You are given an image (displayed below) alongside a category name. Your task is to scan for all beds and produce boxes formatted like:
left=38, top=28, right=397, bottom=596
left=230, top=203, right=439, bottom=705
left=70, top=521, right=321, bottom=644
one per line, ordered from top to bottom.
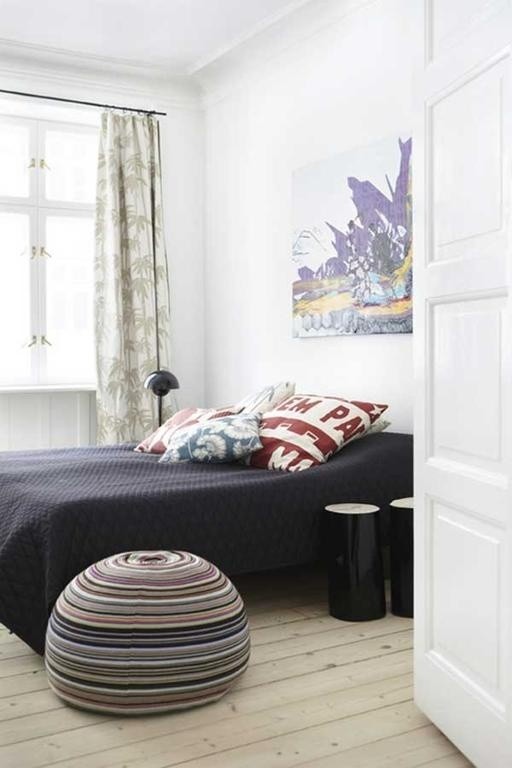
left=0, top=424, right=413, bottom=651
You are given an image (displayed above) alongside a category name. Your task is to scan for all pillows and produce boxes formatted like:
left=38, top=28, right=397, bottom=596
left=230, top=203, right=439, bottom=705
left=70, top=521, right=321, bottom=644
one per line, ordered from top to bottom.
left=233, top=391, right=388, bottom=471
left=135, top=405, right=243, bottom=455
left=159, top=411, right=262, bottom=463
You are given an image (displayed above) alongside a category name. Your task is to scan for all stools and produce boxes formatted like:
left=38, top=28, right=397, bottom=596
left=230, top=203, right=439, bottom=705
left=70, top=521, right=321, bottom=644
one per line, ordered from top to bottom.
left=45, top=548, right=252, bottom=718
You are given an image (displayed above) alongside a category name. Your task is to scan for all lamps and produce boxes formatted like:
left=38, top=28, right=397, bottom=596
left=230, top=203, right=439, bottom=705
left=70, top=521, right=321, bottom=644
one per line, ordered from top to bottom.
left=143, top=370, right=179, bottom=427
